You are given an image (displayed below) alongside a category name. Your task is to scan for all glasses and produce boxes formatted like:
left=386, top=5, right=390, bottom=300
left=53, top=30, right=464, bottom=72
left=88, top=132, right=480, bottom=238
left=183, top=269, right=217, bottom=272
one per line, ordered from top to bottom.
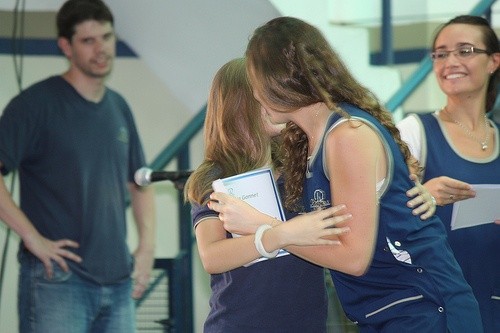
left=430, top=46, right=489, bottom=62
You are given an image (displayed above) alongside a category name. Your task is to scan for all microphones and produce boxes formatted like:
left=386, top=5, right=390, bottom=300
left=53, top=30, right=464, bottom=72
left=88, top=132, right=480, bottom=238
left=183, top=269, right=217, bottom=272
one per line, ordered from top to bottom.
left=134, top=167, right=194, bottom=187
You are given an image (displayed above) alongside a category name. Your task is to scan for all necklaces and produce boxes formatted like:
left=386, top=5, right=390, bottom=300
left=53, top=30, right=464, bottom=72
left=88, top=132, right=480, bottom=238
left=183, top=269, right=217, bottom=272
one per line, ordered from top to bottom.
left=306, top=102, right=325, bottom=160
left=443, top=105, right=492, bottom=151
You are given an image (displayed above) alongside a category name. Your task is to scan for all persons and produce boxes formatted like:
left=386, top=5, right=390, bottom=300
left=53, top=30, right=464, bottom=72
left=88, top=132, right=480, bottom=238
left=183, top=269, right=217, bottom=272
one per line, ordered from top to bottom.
left=0, top=0, right=160, bottom=333
left=395, top=15, right=500, bottom=333
left=205, top=16, right=484, bottom=332
left=182, top=58, right=438, bottom=333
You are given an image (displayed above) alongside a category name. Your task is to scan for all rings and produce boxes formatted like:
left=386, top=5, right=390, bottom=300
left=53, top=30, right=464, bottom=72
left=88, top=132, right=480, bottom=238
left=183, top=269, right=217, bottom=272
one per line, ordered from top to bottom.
left=450, top=194, right=456, bottom=203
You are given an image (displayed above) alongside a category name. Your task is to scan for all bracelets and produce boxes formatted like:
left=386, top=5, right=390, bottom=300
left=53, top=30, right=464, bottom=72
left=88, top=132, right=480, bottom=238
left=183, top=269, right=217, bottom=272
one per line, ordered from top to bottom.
left=270, top=216, right=277, bottom=227
left=254, top=223, right=280, bottom=259
